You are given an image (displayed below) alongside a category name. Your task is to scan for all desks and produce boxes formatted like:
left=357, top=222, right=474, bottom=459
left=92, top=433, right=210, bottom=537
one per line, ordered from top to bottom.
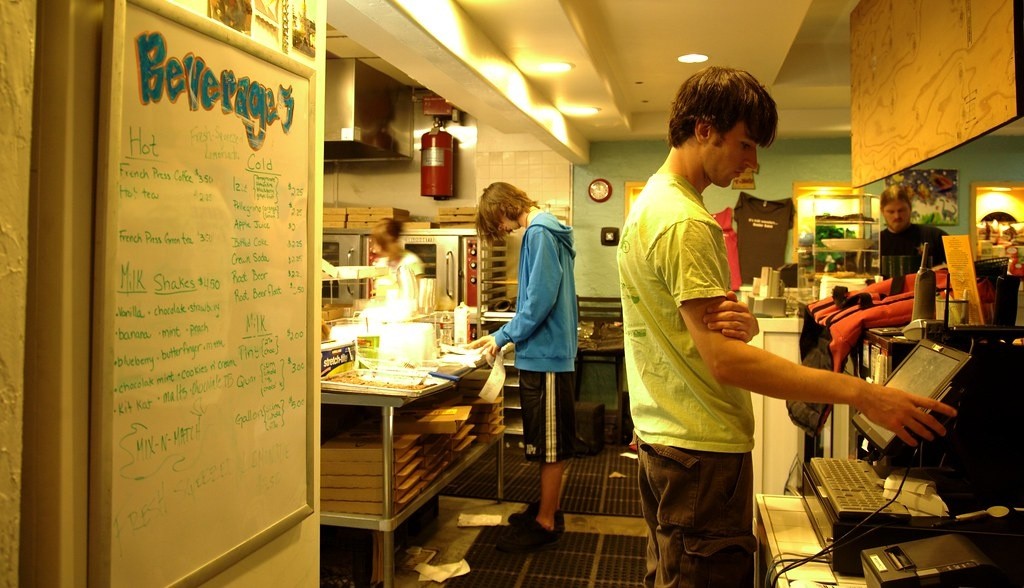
left=320, top=341, right=516, bottom=588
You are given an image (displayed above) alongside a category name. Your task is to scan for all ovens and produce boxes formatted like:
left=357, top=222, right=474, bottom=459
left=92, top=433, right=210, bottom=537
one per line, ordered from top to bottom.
left=323, top=227, right=499, bottom=343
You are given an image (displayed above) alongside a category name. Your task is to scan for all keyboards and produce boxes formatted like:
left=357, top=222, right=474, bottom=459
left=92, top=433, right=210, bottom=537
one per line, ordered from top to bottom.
left=810, top=458, right=911, bottom=523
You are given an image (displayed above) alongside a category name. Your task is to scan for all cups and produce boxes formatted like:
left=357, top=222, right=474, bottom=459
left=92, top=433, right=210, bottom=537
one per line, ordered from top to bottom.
left=991, top=275, right=1021, bottom=327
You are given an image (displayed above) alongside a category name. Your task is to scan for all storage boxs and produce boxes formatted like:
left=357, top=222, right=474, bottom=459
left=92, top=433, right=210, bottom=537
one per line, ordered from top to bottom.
left=320, top=368, right=507, bottom=514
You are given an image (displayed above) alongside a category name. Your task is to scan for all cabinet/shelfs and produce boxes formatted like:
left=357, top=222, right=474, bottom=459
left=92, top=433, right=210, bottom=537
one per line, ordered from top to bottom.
left=795, top=194, right=881, bottom=291
left=477, top=203, right=571, bottom=448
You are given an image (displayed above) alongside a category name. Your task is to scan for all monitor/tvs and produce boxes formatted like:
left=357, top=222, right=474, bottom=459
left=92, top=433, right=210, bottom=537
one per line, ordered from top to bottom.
left=852, top=339, right=973, bottom=457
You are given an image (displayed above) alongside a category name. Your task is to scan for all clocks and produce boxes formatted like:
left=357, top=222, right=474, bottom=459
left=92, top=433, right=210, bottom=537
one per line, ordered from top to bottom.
left=589, top=179, right=612, bottom=202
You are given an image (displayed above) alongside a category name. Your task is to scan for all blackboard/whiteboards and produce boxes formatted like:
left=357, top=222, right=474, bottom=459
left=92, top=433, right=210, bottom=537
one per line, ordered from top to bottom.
left=93, top=0, right=320, bottom=588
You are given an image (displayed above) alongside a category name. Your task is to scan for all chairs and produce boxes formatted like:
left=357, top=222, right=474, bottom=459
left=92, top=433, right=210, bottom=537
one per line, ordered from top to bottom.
left=572, top=294, right=626, bottom=398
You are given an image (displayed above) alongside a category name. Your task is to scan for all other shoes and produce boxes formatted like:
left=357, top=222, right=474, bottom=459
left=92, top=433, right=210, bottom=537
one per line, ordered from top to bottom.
left=508, top=506, right=564, bottom=534
left=496, top=517, right=561, bottom=555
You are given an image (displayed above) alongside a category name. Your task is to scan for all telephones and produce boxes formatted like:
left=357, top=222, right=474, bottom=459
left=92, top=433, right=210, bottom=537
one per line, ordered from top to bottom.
left=904, top=241, right=946, bottom=340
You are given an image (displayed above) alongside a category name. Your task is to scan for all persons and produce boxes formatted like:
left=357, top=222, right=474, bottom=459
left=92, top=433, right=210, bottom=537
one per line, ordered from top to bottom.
left=616, top=68, right=956, bottom=588
left=464, top=182, right=579, bottom=553
left=869, top=184, right=950, bottom=272
left=371, top=220, right=425, bottom=310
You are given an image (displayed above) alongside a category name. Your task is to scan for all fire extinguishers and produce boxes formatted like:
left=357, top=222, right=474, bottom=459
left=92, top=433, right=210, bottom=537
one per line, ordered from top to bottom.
left=417, top=113, right=455, bottom=199
